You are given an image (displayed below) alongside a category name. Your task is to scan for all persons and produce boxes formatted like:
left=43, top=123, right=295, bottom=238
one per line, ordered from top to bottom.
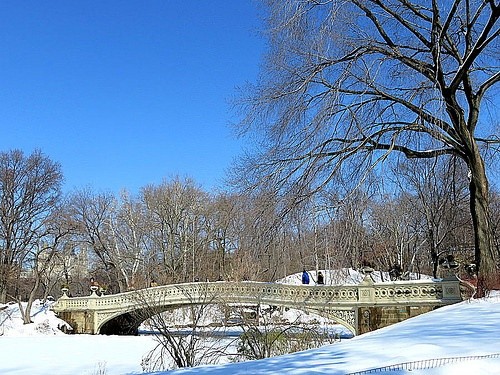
left=302, top=270, right=309, bottom=284
left=318, top=272, right=324, bottom=284
